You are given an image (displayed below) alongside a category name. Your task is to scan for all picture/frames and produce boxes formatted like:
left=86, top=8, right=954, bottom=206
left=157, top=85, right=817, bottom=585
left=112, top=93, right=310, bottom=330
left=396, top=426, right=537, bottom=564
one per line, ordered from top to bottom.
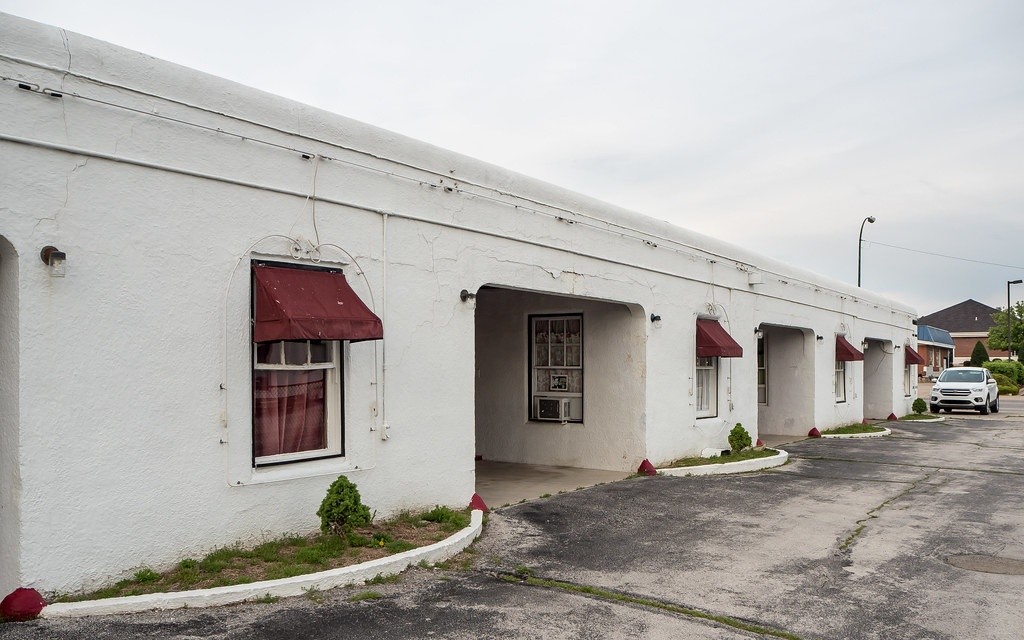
left=550, top=375, right=568, bottom=391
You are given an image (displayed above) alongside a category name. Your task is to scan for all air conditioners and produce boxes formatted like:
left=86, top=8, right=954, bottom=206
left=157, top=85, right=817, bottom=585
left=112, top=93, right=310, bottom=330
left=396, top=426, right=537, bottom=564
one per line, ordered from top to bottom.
left=537, top=398, right=571, bottom=420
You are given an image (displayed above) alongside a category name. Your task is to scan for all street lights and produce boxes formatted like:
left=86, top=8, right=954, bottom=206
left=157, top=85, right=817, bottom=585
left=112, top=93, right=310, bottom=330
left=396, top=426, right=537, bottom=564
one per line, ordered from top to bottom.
left=857, top=216, right=876, bottom=287
left=1007, top=280, right=1023, bottom=364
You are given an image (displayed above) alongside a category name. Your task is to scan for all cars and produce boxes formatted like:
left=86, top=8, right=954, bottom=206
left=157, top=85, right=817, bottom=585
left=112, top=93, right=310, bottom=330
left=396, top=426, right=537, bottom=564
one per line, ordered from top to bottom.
left=930, top=366, right=1000, bottom=415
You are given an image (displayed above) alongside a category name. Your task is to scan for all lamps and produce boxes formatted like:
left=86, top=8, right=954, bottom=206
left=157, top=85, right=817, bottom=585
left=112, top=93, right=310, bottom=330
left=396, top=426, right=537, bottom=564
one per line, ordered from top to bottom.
left=42, top=246, right=68, bottom=278
left=461, top=289, right=476, bottom=313
left=650, top=314, right=660, bottom=328
left=754, top=326, right=764, bottom=338
left=817, top=334, right=824, bottom=345
left=861, top=341, right=869, bottom=350
left=894, top=345, right=902, bottom=353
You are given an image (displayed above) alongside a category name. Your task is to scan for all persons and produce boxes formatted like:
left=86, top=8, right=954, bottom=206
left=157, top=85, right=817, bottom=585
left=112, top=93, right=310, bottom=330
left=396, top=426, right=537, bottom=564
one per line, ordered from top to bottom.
left=552, top=378, right=563, bottom=389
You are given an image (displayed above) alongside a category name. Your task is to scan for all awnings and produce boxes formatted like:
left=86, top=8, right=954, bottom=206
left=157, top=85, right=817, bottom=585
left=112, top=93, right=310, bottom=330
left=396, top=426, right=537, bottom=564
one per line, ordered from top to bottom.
left=252, top=264, right=385, bottom=342
left=697, top=319, right=744, bottom=358
left=836, top=336, right=865, bottom=361
left=905, top=346, right=925, bottom=364
